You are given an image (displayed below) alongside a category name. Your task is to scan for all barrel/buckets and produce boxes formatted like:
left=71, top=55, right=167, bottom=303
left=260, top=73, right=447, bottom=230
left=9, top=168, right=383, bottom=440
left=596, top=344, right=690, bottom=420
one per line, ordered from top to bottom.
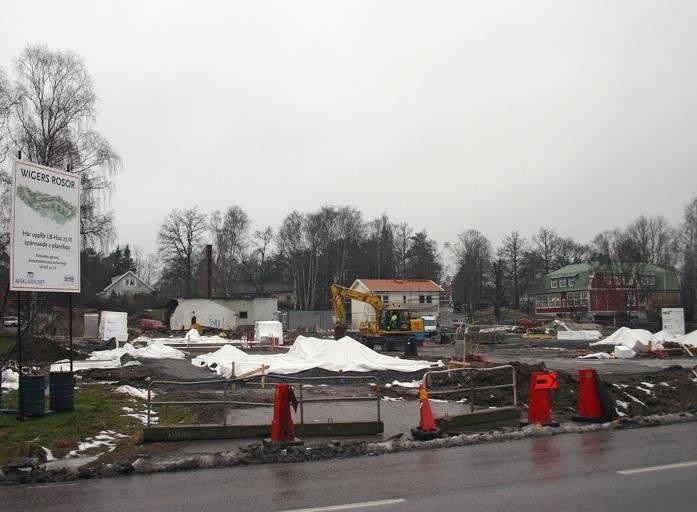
left=85, top=314, right=98, bottom=338
left=455, top=339, right=473, bottom=359
left=49, top=371, right=74, bottom=411
left=19, top=373, right=45, bottom=417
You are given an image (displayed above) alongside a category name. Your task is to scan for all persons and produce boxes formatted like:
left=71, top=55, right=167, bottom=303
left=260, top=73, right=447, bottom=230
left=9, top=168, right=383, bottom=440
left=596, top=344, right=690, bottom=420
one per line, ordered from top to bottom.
left=191, top=309, right=196, bottom=325
left=390, top=310, right=397, bottom=322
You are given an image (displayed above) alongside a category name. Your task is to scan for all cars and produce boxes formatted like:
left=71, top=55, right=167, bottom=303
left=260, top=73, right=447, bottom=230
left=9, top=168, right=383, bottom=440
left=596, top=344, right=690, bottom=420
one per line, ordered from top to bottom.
left=51, top=305, right=100, bottom=317
left=138, top=317, right=166, bottom=332
left=478, top=324, right=554, bottom=335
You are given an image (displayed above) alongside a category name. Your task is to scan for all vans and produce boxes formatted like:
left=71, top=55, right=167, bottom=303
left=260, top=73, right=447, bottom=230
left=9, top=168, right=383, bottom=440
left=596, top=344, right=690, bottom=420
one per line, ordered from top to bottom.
left=418, top=315, right=443, bottom=336
left=3, top=316, right=27, bottom=326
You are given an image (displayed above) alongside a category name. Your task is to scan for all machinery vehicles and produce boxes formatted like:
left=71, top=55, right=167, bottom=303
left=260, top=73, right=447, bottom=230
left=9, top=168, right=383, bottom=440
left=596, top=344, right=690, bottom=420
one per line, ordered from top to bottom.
left=328, top=278, right=426, bottom=355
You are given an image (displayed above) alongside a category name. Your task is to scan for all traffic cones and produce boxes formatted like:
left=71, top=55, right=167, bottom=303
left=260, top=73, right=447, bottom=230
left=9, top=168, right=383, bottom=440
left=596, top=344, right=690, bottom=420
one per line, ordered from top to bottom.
left=572, top=365, right=607, bottom=424
left=518, top=369, right=562, bottom=427
left=261, top=382, right=304, bottom=450
left=414, top=383, right=439, bottom=433
